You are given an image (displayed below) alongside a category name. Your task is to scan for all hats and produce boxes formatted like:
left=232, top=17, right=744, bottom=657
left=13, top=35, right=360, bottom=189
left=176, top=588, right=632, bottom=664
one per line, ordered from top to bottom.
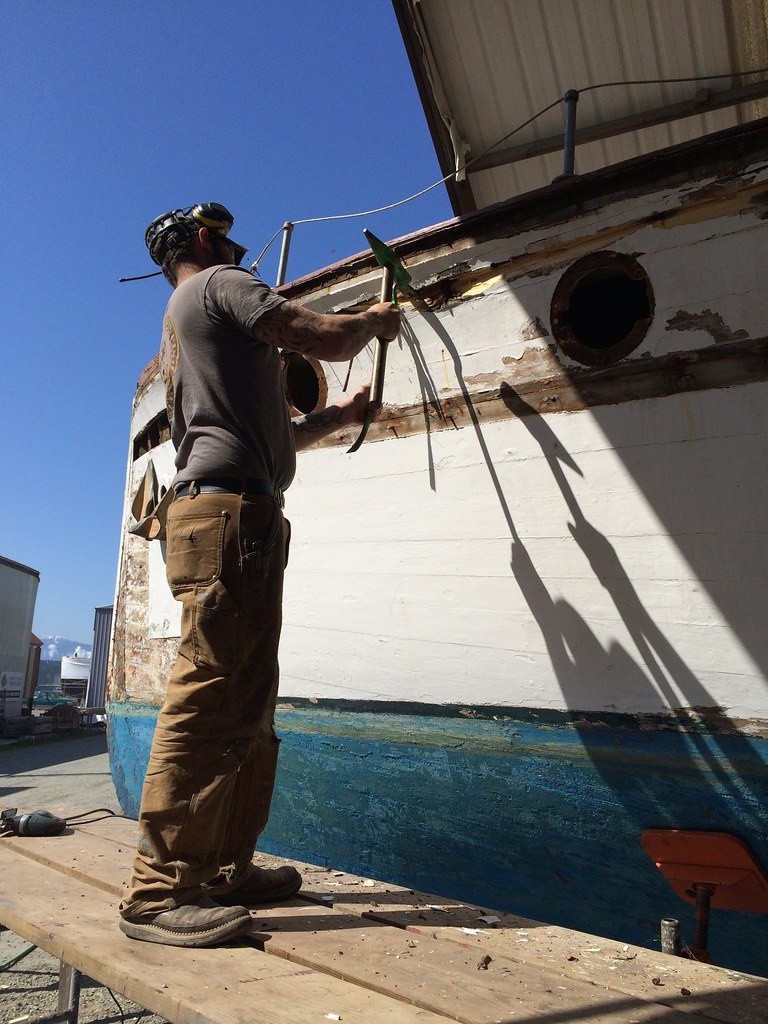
left=146, top=214, right=248, bottom=267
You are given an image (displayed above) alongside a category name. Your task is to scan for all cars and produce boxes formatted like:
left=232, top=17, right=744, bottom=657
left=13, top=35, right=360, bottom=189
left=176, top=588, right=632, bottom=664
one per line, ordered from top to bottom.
left=22, top=690, right=76, bottom=711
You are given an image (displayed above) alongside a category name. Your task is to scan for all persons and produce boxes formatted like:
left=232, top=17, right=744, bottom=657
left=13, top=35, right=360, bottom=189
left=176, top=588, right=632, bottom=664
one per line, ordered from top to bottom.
left=116, top=201, right=400, bottom=947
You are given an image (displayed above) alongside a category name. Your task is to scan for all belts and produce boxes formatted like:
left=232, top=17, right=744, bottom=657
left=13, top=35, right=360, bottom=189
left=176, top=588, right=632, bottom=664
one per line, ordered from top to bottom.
left=172, top=477, right=285, bottom=510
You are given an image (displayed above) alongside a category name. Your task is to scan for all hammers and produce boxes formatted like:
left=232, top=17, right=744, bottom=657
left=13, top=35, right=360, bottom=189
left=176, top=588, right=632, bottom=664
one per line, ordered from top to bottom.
left=362, top=227, right=413, bottom=410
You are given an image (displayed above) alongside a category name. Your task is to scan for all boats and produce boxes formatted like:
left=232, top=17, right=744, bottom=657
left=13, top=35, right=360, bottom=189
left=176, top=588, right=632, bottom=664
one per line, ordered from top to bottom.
left=105, top=2, right=767, bottom=980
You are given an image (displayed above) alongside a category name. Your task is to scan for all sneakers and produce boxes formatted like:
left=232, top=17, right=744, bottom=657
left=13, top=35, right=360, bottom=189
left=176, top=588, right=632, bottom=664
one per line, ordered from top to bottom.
left=205, top=865, right=303, bottom=906
left=121, top=900, right=255, bottom=946
